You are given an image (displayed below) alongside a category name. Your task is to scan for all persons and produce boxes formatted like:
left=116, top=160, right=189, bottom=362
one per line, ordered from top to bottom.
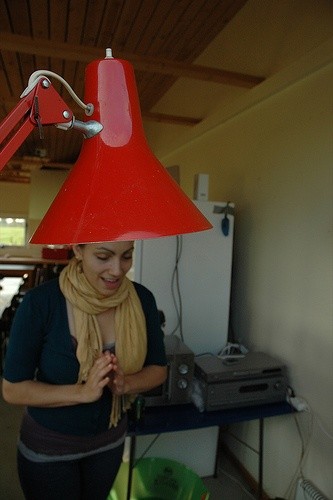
left=0, top=241, right=168, bottom=500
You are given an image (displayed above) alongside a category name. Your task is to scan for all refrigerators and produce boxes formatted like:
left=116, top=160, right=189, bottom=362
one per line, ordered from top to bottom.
left=121, top=201, right=235, bottom=477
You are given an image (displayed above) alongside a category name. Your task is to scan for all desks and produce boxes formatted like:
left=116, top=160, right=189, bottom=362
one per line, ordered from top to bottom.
left=125, top=401, right=304, bottom=500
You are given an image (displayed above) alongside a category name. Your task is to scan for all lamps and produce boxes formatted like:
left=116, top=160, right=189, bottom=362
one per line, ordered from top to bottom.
left=0, top=48, right=213, bottom=245
